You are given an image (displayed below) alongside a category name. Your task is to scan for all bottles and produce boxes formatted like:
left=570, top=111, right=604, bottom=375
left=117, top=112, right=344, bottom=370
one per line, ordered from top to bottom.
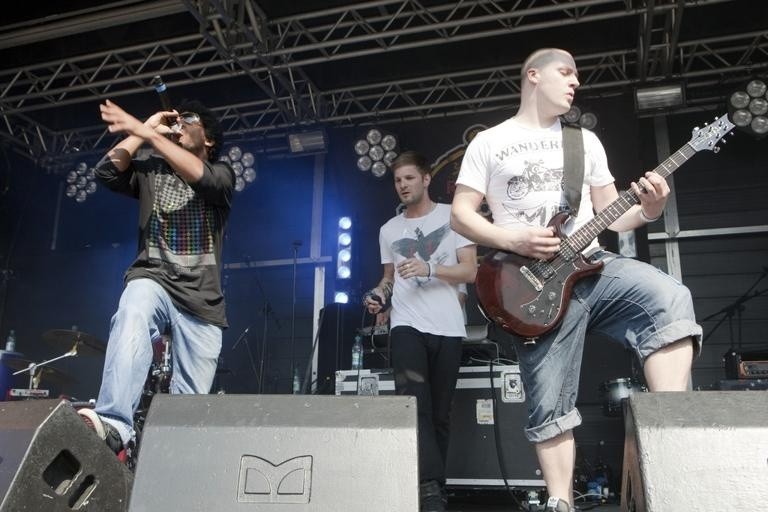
left=5, top=330, right=17, bottom=352
left=350, top=336, right=365, bottom=370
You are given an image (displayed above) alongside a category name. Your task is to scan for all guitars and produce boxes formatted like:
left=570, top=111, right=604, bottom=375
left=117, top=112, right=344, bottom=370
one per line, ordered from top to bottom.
left=475, top=113, right=737, bottom=345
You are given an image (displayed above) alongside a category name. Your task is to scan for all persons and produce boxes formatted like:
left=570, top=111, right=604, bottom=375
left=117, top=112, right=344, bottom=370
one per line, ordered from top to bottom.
left=446, top=45, right=704, bottom=511
left=75, top=97, right=239, bottom=465
left=358, top=147, right=481, bottom=511
left=452, top=281, right=470, bottom=329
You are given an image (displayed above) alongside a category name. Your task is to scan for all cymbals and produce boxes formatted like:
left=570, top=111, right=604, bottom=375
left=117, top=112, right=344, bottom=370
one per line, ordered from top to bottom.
left=41, top=329, right=107, bottom=361
left=4, top=357, right=81, bottom=388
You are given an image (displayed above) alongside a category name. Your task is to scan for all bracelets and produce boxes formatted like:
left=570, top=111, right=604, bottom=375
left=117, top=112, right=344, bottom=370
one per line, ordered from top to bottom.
left=638, top=210, right=663, bottom=224
left=426, top=261, right=433, bottom=280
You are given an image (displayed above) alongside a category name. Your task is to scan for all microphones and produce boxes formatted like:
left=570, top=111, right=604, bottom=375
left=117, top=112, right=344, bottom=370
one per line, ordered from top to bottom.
left=153, top=71, right=183, bottom=138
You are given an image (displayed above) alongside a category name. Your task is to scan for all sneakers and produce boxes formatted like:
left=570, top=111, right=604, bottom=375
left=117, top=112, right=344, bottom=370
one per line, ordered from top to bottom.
left=78, top=407, right=124, bottom=455
left=421, top=481, right=447, bottom=512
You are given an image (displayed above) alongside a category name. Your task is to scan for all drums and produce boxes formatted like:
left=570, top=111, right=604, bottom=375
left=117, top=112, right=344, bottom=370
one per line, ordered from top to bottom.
left=150, top=334, right=172, bottom=384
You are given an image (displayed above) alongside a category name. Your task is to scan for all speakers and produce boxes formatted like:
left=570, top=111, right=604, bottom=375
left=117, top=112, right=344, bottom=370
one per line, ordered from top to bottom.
left=620, top=387, right=768, bottom=512
left=128, top=394, right=422, bottom=512
left=0, top=397, right=134, bottom=512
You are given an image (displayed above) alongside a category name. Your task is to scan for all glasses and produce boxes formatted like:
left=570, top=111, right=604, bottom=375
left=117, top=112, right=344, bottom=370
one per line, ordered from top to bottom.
left=179, top=112, right=204, bottom=128
